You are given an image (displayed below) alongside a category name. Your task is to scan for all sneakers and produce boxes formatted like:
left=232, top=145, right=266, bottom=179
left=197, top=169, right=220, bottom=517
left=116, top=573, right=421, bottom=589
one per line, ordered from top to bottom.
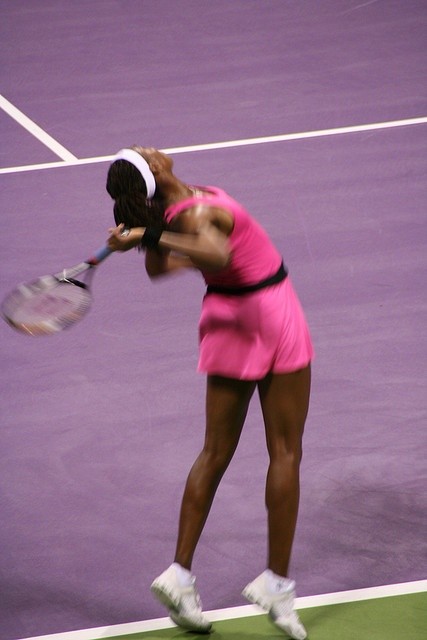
left=149, top=568, right=212, bottom=632
left=241, top=571, right=307, bottom=640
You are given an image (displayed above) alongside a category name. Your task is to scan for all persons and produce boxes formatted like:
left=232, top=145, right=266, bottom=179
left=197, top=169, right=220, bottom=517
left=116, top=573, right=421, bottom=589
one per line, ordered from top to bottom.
left=107, top=146, right=314, bottom=640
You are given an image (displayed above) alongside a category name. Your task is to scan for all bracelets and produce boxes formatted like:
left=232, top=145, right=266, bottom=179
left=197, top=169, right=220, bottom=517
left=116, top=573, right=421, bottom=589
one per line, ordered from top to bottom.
left=140, top=225, right=166, bottom=251
